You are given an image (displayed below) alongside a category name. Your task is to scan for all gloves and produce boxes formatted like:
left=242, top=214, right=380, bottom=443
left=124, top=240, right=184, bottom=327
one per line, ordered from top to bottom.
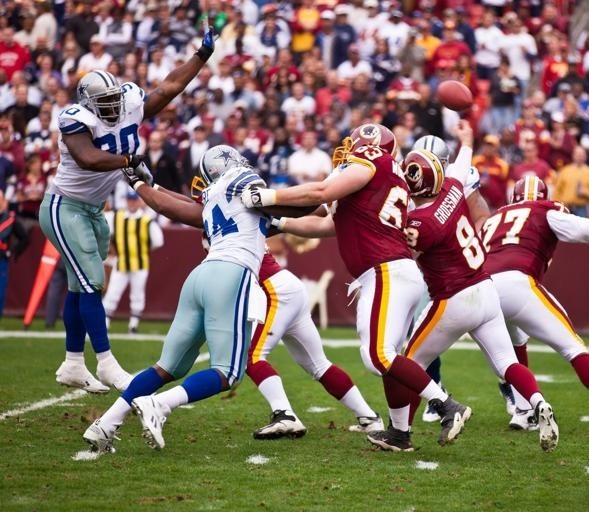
left=194, top=16, right=219, bottom=60
left=121, top=152, right=153, bottom=189
left=241, top=187, right=277, bottom=209
left=265, top=215, right=286, bottom=238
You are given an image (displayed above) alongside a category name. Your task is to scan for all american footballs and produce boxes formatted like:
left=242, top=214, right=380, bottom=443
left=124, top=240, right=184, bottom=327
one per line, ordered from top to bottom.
left=437, top=80, right=473, bottom=111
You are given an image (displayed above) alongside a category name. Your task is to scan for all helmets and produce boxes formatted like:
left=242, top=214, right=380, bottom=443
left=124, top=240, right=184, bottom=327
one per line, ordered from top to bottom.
left=78, top=70, right=126, bottom=125
left=333, top=123, right=450, bottom=197
left=201, top=145, right=248, bottom=183
left=514, top=175, right=547, bottom=202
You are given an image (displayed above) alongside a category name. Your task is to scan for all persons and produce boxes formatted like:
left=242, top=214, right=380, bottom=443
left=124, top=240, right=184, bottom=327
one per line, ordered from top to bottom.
left=1, top=0, right=589, bottom=224
left=37, top=27, right=219, bottom=394
left=393, top=119, right=562, bottom=454
left=241, top=123, right=472, bottom=454
left=407, top=135, right=519, bottom=423
left=84, top=144, right=345, bottom=450
left=124, top=163, right=386, bottom=441
left=472, top=175, right=589, bottom=432
left=104, top=188, right=164, bottom=332
left=0, top=191, right=29, bottom=316
left=44, top=259, right=68, bottom=327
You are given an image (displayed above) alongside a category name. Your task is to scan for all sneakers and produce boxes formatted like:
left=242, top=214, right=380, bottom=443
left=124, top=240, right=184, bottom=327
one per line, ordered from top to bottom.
left=96, top=357, right=133, bottom=392
left=56, top=362, right=111, bottom=393
left=497, top=382, right=559, bottom=454
left=349, top=388, right=471, bottom=454
left=131, top=395, right=165, bottom=450
left=253, top=410, right=307, bottom=438
left=82, top=419, right=116, bottom=454
